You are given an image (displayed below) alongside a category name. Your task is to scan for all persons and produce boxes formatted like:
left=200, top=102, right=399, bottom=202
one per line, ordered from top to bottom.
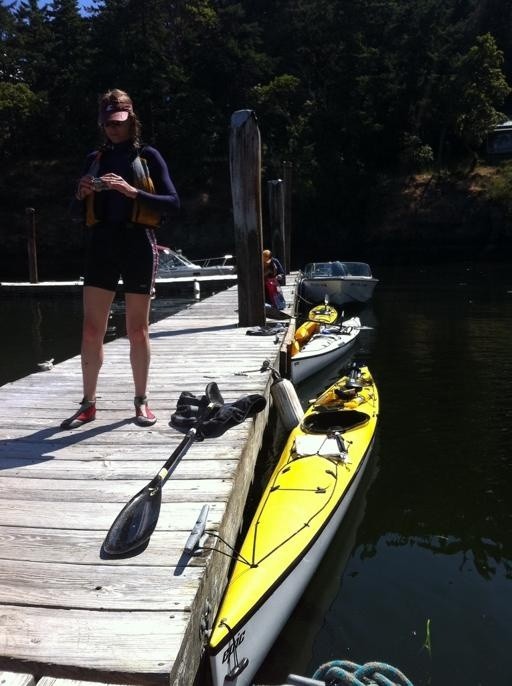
left=58, top=87, right=182, bottom=431
left=263, top=250, right=285, bottom=305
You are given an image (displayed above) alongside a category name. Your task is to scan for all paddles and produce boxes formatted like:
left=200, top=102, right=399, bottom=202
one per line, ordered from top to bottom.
left=324, top=294, right=329, bottom=312
left=305, top=318, right=373, bottom=330
left=101, top=382, right=223, bottom=553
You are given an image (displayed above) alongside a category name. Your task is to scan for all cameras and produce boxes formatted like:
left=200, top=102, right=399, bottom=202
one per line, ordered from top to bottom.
left=92, top=178, right=108, bottom=192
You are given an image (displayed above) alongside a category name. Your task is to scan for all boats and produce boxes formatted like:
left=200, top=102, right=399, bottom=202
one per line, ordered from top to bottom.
left=156, top=244, right=236, bottom=278
left=301, top=261, right=380, bottom=307
left=292, top=304, right=362, bottom=385
left=203, top=364, right=381, bottom=686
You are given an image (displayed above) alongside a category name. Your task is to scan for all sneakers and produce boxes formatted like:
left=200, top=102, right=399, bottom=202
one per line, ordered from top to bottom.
left=133, top=396, right=158, bottom=426
left=59, top=397, right=97, bottom=429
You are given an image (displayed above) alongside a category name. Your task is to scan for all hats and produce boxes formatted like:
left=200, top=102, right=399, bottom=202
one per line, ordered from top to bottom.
left=96, top=99, right=133, bottom=127
left=263, top=249, right=272, bottom=265
